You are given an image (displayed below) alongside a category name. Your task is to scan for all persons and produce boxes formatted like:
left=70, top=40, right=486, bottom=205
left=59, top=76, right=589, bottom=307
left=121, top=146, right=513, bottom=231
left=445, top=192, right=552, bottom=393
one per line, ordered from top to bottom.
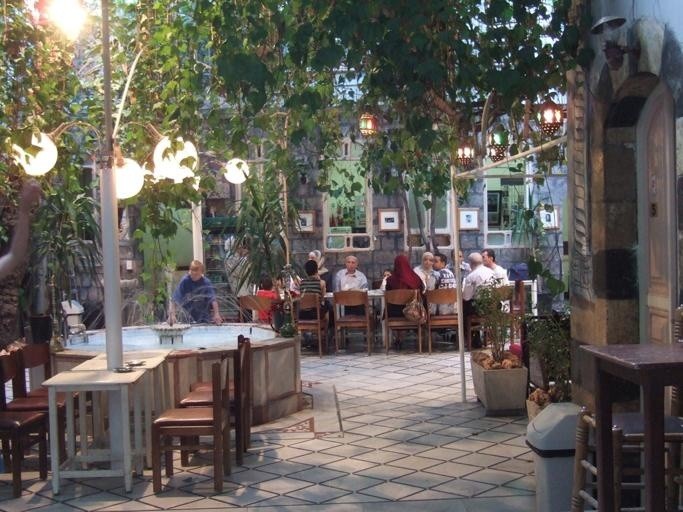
left=222, top=235, right=254, bottom=322
left=308, top=249, right=331, bottom=287
left=379, top=249, right=512, bottom=353
left=332, top=255, right=367, bottom=314
left=165, top=260, right=224, bottom=327
left=258, top=260, right=329, bottom=347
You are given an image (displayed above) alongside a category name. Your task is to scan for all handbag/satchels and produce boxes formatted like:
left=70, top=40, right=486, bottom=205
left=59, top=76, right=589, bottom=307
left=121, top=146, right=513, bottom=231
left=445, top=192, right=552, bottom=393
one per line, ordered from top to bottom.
left=403, top=290, right=428, bottom=325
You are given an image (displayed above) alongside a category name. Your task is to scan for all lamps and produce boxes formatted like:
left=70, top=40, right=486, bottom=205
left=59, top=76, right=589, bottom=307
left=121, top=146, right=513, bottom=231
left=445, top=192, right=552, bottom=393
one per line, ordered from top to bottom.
left=591, top=16, right=641, bottom=71
left=538, top=93, right=564, bottom=138
left=357, top=113, right=378, bottom=137
left=486, top=133, right=505, bottom=163
left=458, top=141, right=474, bottom=167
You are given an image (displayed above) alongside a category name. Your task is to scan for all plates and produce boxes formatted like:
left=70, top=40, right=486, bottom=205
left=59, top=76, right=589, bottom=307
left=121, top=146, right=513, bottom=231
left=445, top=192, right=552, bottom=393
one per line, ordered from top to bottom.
left=126, top=361, right=146, bottom=366
left=112, top=368, right=134, bottom=372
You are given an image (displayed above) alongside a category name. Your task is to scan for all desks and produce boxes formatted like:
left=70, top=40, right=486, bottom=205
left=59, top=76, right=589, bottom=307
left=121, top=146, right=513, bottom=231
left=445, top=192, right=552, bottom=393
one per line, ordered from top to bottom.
left=576, top=342, right=683, bottom=512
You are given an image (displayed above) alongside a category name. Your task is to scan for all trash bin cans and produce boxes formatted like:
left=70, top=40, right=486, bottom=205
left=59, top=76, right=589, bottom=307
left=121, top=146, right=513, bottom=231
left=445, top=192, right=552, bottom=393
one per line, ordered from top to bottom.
left=525, top=402, right=593, bottom=512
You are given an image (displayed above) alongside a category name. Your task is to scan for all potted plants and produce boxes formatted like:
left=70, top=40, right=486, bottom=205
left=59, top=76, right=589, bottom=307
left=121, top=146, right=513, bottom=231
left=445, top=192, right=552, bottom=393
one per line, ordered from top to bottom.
left=470, top=277, right=530, bottom=411
left=526, top=317, right=571, bottom=425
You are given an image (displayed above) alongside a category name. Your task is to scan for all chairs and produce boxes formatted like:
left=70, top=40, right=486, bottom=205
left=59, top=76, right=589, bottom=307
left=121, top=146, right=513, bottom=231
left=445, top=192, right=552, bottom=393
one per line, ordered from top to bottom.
left=238, top=269, right=527, bottom=358
left=571, top=302, right=683, bottom=512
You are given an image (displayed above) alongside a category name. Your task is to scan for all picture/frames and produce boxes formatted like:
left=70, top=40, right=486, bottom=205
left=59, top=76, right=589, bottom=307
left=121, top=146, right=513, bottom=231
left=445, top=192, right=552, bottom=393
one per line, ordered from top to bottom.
left=488, top=190, right=502, bottom=226
left=538, top=205, right=560, bottom=230
left=378, top=208, right=402, bottom=233
left=457, top=208, right=479, bottom=232
left=293, top=210, right=316, bottom=234
